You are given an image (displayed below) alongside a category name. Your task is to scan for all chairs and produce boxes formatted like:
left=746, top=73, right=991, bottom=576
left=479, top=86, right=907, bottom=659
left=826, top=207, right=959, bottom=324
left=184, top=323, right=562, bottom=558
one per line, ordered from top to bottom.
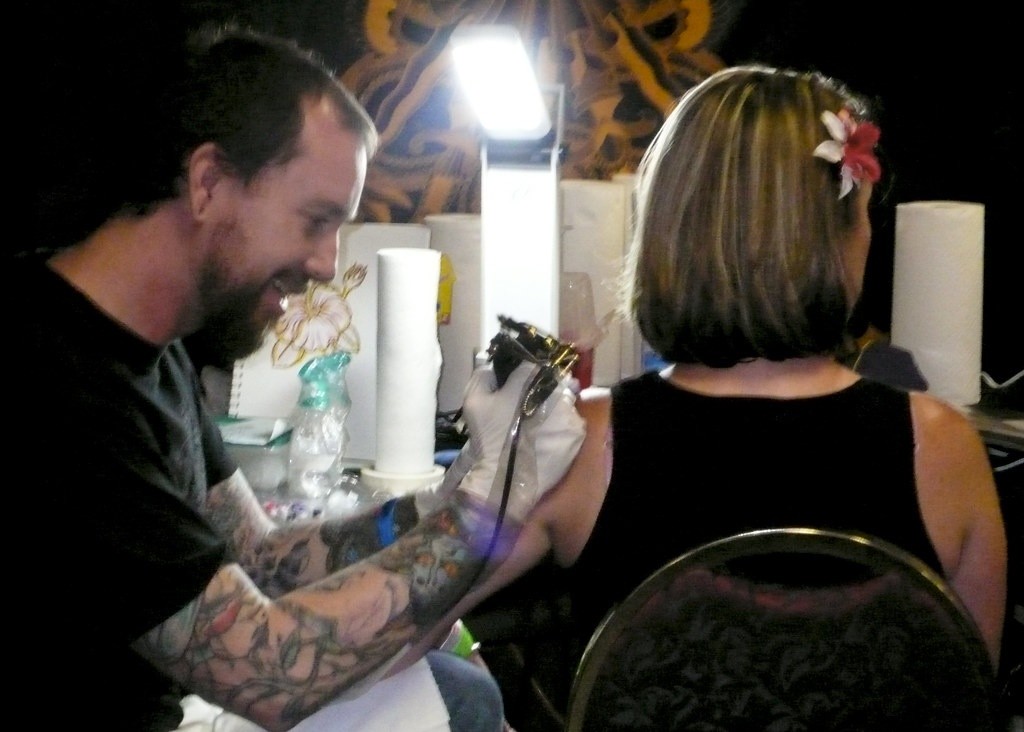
left=470, top=528, right=1014, bottom=732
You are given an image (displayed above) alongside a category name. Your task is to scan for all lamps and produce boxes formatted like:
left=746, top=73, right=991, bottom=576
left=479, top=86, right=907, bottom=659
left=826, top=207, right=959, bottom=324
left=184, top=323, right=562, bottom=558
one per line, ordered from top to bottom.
left=447, top=20, right=566, bottom=345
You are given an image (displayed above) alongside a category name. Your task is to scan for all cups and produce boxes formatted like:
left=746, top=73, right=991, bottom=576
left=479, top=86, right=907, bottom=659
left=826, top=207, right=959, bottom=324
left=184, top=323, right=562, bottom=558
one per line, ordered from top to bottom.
left=558, top=272, right=597, bottom=389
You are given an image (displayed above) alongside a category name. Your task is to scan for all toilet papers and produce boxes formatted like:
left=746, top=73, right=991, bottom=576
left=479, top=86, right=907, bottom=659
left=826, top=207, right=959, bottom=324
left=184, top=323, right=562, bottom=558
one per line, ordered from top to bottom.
left=557, top=170, right=651, bottom=388
left=890, top=200, right=985, bottom=405
left=422, top=211, right=481, bottom=414
left=370, top=246, right=444, bottom=476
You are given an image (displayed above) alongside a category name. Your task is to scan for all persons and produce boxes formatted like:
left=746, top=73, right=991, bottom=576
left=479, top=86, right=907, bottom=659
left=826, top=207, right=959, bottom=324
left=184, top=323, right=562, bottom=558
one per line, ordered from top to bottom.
left=0, top=24, right=587, bottom=732
left=381, top=67, right=1009, bottom=732
